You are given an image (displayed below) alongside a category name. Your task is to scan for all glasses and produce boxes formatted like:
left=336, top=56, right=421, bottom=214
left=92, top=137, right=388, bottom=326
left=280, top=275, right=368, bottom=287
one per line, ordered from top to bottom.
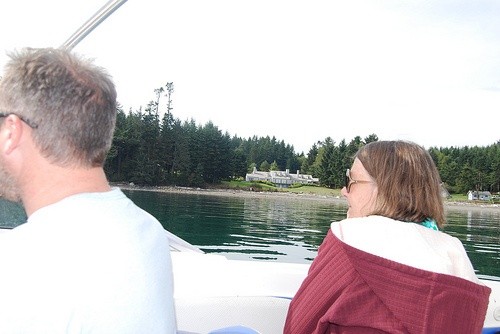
left=346, top=169, right=374, bottom=193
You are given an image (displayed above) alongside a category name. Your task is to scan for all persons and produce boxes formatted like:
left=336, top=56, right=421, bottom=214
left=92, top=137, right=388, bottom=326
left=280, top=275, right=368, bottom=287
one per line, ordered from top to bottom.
left=0, top=48, right=176, bottom=334
left=282, top=141, right=491, bottom=334
